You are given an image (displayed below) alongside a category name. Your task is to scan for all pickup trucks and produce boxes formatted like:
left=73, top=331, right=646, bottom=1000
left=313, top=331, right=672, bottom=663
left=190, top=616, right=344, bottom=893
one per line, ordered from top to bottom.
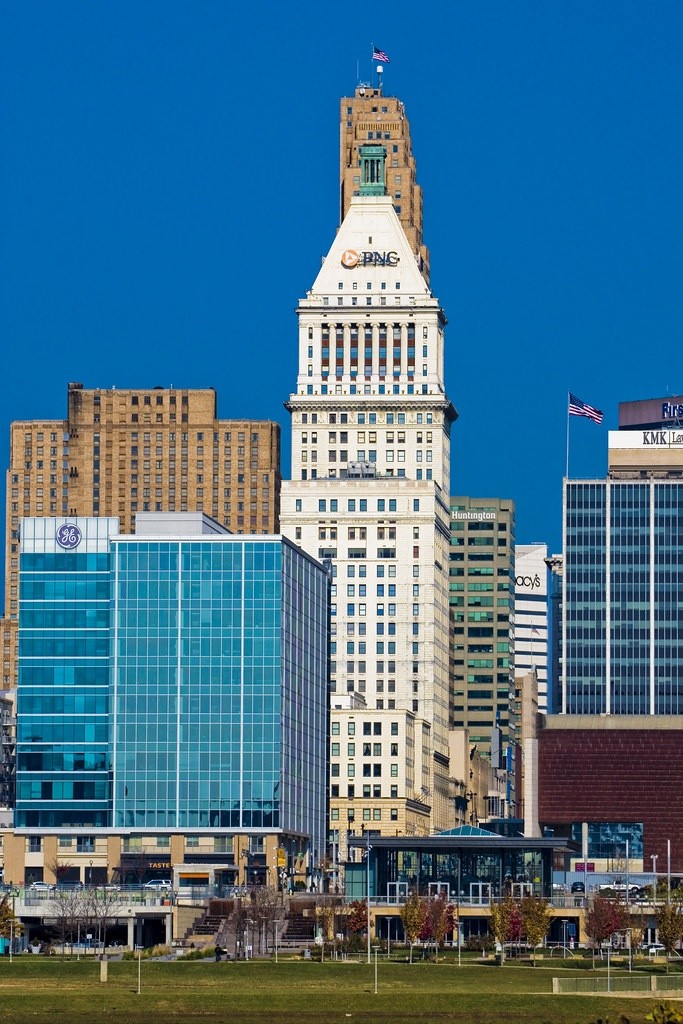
left=599, top=881, right=643, bottom=893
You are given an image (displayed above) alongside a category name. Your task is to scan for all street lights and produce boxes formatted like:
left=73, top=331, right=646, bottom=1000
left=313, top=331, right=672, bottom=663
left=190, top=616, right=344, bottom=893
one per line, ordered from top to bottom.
left=362, top=829, right=372, bottom=963
left=650, top=854, right=658, bottom=909
left=89, top=860, right=94, bottom=889
left=6, top=885, right=21, bottom=937
left=451, top=889, right=464, bottom=948
left=165, top=891, right=179, bottom=953
left=400, top=892, right=414, bottom=942
left=385, top=916, right=392, bottom=959
left=261, top=917, right=266, bottom=954
left=76, top=918, right=83, bottom=960
left=244, top=918, right=251, bottom=961
left=6, top=919, right=15, bottom=963
left=273, top=919, right=280, bottom=963
left=561, top=920, right=569, bottom=959
left=372, top=945, right=380, bottom=994
left=136, top=946, right=144, bottom=995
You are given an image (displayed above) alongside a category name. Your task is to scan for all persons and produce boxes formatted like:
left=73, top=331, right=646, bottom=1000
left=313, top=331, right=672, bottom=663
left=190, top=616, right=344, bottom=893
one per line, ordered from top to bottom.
left=215, top=944, right=221, bottom=962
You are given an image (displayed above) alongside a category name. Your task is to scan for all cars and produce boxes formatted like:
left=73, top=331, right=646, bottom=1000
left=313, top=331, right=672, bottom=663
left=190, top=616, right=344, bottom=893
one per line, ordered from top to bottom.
left=140, top=878, right=175, bottom=891
left=571, top=881, right=585, bottom=893
left=29, top=882, right=56, bottom=891
left=93, top=883, right=121, bottom=892
left=0, top=884, right=21, bottom=897
left=68, top=938, right=124, bottom=948
left=646, top=943, right=666, bottom=951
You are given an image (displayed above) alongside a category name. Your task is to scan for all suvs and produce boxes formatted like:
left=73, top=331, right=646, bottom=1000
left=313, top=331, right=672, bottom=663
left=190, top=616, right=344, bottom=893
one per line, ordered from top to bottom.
left=54, top=880, right=84, bottom=890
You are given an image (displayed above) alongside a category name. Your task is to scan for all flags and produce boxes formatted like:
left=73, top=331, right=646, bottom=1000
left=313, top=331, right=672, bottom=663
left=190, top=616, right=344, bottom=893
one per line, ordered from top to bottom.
left=373, top=47, right=390, bottom=63
left=569, top=393, right=604, bottom=424
